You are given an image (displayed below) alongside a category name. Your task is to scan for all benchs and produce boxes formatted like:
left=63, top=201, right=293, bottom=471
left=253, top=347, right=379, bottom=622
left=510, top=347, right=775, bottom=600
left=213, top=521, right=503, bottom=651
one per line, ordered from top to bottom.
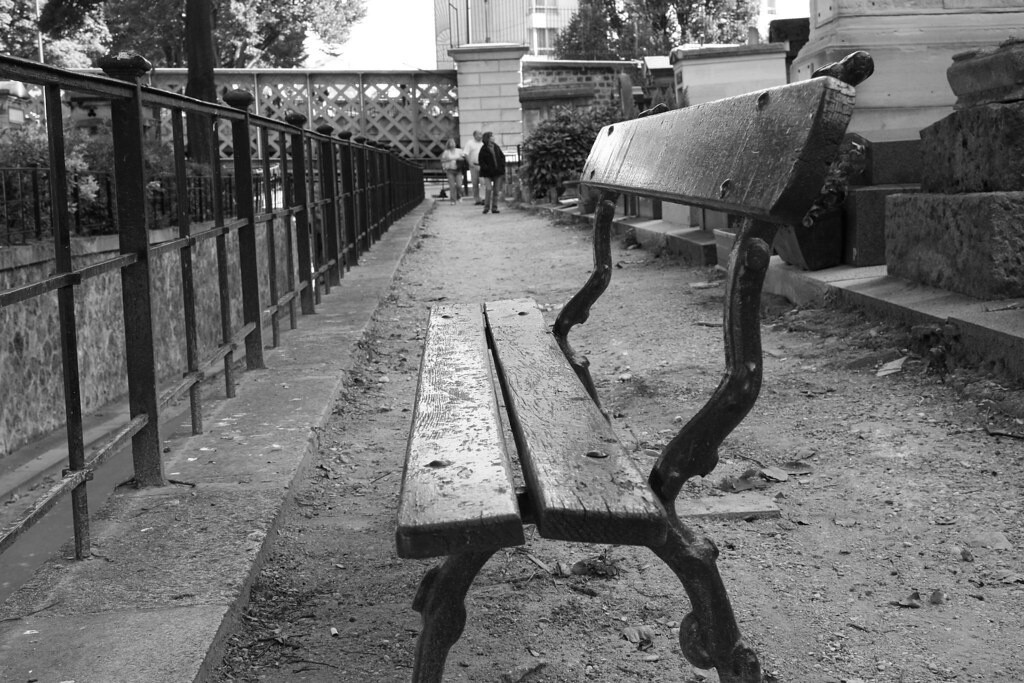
left=398, top=49, right=877, bottom=683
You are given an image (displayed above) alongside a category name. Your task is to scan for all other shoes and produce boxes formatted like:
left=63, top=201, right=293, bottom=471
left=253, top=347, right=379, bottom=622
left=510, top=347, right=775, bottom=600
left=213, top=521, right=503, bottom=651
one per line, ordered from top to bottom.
left=458, top=198, right=462, bottom=203
left=481, top=199, right=485, bottom=205
left=451, top=202, right=455, bottom=205
left=474, top=202, right=481, bottom=205
left=483, top=209, right=488, bottom=213
left=492, top=211, right=499, bottom=213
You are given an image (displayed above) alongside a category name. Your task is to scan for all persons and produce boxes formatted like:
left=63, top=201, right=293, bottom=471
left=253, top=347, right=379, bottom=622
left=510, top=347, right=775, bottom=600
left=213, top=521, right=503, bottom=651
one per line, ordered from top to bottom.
left=463, top=130, right=485, bottom=205
left=477, top=131, right=506, bottom=214
left=440, top=138, right=464, bottom=206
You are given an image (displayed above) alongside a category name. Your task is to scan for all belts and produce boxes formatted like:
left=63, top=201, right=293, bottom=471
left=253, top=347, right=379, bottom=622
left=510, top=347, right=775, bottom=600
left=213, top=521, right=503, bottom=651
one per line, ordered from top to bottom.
left=473, top=163, right=481, bottom=166
